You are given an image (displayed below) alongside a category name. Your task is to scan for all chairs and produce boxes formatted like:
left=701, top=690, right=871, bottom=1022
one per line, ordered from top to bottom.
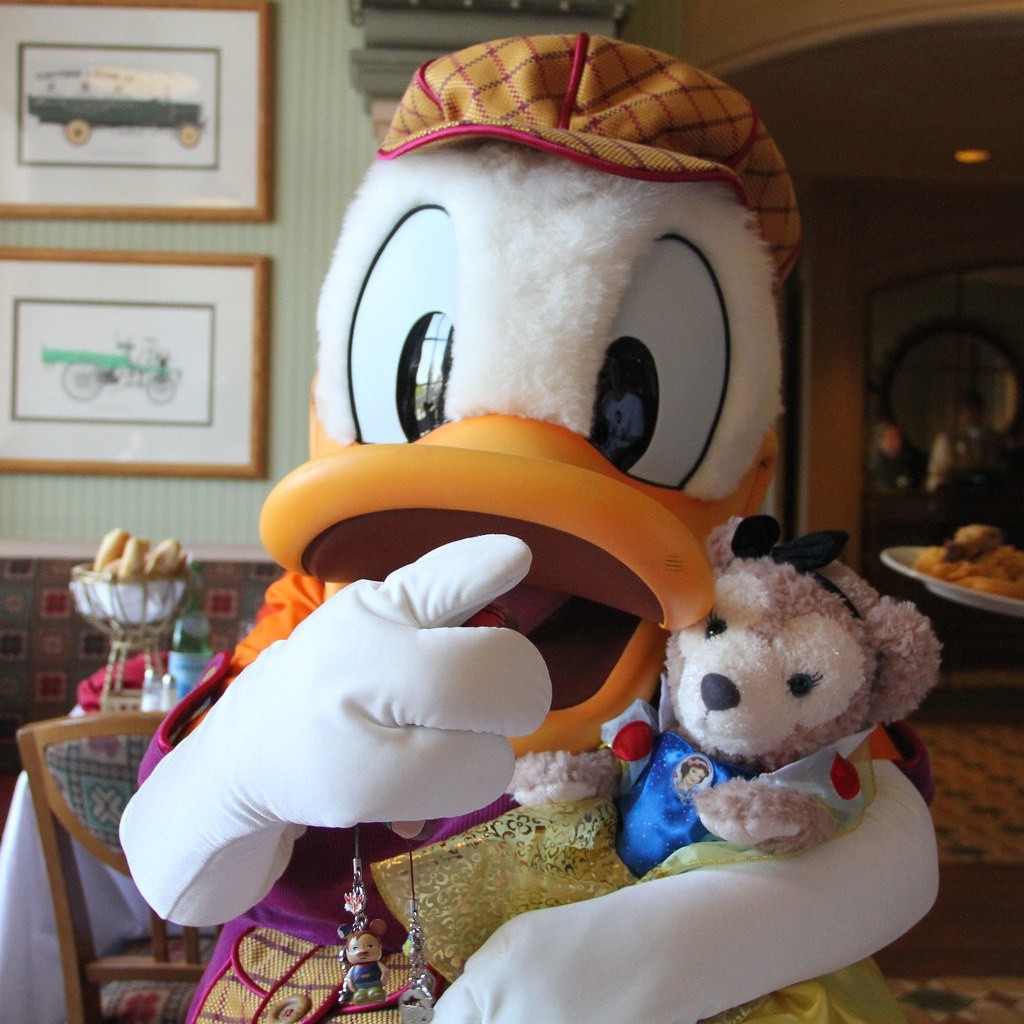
left=16, top=704, right=226, bottom=1023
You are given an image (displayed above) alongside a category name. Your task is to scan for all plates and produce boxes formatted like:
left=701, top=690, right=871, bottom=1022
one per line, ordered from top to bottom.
left=879, top=545, right=1024, bottom=619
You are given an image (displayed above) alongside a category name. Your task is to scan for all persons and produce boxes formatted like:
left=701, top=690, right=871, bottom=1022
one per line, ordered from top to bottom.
left=599, top=355, right=644, bottom=456
left=926, top=390, right=1008, bottom=489
left=864, top=420, right=927, bottom=491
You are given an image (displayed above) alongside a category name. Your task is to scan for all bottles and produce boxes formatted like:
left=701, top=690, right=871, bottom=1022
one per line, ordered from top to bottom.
left=168, top=559, right=213, bottom=710
left=140, top=668, right=158, bottom=711
left=159, top=674, right=175, bottom=710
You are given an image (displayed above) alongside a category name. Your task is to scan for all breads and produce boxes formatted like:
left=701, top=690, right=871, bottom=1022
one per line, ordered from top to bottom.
left=913, top=525, right=1024, bottom=600
left=87, top=528, right=188, bottom=581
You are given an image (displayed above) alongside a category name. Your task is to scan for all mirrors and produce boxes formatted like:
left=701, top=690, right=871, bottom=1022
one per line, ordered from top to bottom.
left=882, top=318, right=1022, bottom=459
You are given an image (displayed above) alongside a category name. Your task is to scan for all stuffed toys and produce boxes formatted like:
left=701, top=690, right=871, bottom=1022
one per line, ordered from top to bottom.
left=119, top=33, right=939, bottom=1024
left=503, top=514, right=939, bottom=879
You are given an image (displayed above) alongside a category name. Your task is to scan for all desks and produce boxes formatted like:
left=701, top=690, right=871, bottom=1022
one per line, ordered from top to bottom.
left=861, top=488, right=1024, bottom=580
left=0, top=703, right=255, bottom=1024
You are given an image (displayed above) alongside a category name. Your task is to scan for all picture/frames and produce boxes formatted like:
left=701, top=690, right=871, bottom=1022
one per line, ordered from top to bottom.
left=0, top=0, right=275, bottom=224
left=1, top=244, right=269, bottom=478
